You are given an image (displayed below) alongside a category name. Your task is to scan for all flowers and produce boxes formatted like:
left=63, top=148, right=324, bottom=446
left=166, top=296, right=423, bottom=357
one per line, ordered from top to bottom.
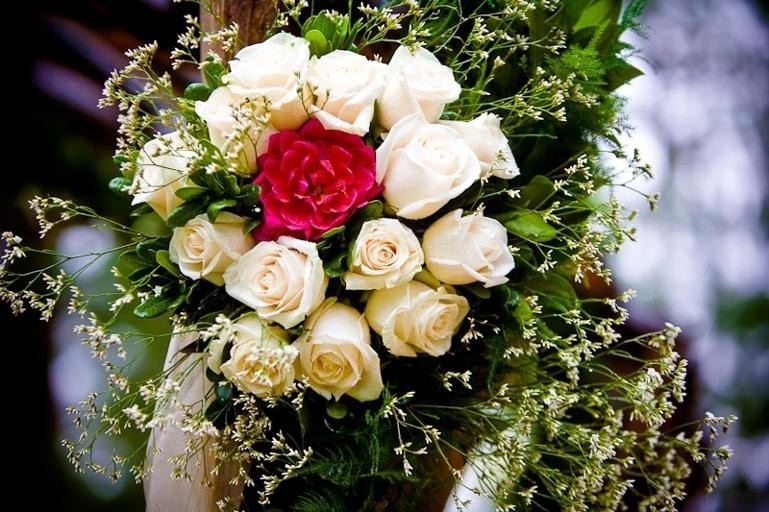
left=0, top=0, right=738, bottom=512
